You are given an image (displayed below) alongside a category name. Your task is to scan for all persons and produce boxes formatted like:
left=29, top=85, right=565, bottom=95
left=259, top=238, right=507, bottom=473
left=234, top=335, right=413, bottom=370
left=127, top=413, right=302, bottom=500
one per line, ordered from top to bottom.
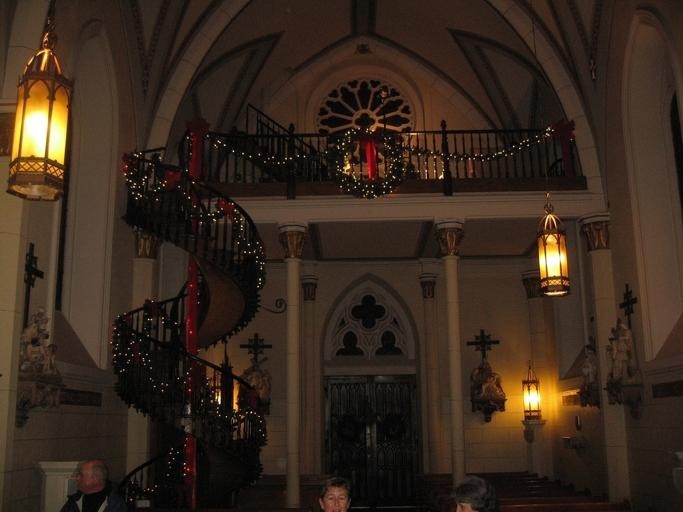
left=60, top=460, right=124, bottom=512
left=318, top=476, right=351, bottom=512
left=449, top=476, right=499, bottom=512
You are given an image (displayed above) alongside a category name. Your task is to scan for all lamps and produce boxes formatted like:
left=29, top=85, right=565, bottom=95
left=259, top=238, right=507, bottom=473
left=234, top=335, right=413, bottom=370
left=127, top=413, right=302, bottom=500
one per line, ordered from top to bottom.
left=6, top=0, right=73, bottom=202
left=535, top=0, right=571, bottom=296
left=522, top=364, right=542, bottom=420
